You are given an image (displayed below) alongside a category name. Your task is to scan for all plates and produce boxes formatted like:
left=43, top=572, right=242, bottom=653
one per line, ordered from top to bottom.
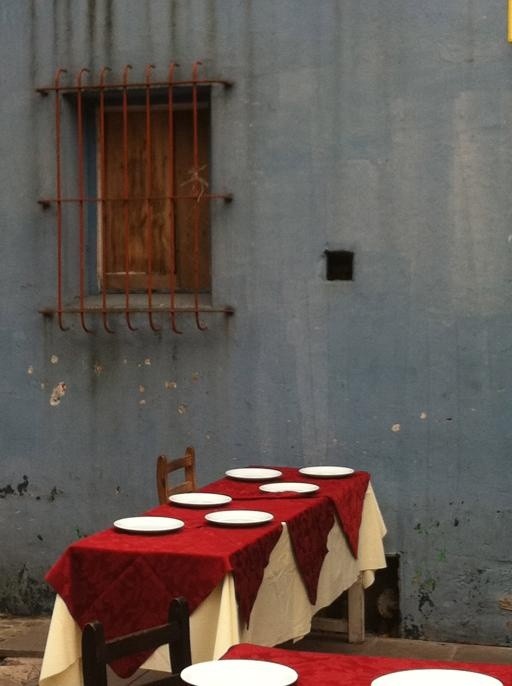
left=296, top=465, right=354, bottom=478
left=226, top=468, right=282, bottom=479
left=257, top=482, right=319, bottom=494
left=169, top=492, right=233, bottom=507
left=204, top=509, right=274, bottom=527
left=113, top=516, right=184, bottom=533
left=180, top=658, right=299, bottom=685
left=368, top=668, right=504, bottom=686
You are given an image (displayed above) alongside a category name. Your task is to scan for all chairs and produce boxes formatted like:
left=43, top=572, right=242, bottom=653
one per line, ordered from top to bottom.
left=155, top=445, right=203, bottom=509
left=81, top=595, right=194, bottom=686
